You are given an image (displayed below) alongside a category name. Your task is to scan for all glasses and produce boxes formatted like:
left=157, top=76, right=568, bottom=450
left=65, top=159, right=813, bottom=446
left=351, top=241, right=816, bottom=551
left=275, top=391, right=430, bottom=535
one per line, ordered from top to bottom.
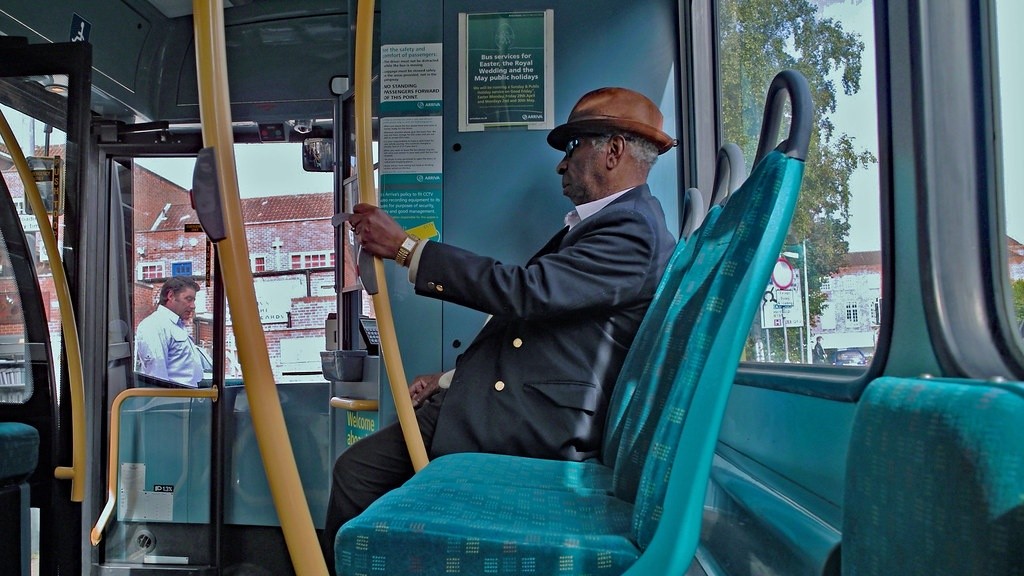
left=565, top=132, right=613, bottom=158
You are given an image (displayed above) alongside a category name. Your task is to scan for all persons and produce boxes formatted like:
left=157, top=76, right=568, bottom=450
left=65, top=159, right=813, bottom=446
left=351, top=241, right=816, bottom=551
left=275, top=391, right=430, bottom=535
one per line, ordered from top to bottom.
left=326, top=87, right=676, bottom=563
left=133, top=278, right=212, bottom=388
left=814, top=337, right=828, bottom=365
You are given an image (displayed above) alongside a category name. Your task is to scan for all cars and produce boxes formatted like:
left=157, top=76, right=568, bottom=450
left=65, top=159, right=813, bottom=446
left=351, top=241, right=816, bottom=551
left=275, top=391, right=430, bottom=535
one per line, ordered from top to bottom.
left=832, top=349, right=868, bottom=366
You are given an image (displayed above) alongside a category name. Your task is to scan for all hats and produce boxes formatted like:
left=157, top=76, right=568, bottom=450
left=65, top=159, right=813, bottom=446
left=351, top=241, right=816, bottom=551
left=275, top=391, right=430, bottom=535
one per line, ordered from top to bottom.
left=547, top=87, right=680, bottom=155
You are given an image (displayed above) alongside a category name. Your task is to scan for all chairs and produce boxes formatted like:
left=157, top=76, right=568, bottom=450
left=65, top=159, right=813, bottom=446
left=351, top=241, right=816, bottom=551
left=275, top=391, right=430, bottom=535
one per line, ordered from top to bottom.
left=841, top=377, right=1023, bottom=576
left=0, top=419, right=40, bottom=576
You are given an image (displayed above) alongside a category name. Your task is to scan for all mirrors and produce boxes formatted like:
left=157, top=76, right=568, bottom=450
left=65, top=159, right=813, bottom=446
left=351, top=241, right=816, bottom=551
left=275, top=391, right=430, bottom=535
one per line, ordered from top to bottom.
left=301, top=136, right=335, bottom=173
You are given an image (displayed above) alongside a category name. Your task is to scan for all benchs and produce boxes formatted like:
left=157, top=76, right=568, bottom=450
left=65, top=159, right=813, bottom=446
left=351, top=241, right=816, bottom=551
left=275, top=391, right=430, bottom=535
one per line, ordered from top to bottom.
left=334, top=71, right=814, bottom=576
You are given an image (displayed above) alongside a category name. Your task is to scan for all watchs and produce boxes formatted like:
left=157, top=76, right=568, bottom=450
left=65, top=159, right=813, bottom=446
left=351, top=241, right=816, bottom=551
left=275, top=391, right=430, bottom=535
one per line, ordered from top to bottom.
left=396, top=235, right=418, bottom=268
